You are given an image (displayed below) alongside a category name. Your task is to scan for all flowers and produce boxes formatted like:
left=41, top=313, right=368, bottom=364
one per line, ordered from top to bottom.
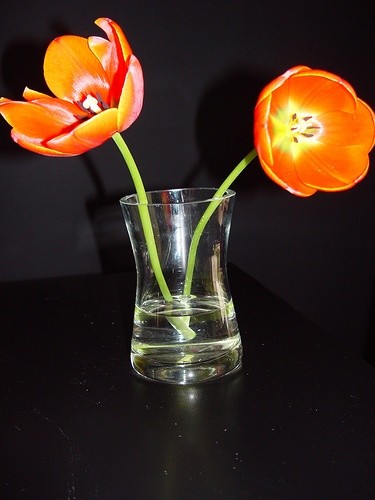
left=0, top=18, right=375, bottom=339
